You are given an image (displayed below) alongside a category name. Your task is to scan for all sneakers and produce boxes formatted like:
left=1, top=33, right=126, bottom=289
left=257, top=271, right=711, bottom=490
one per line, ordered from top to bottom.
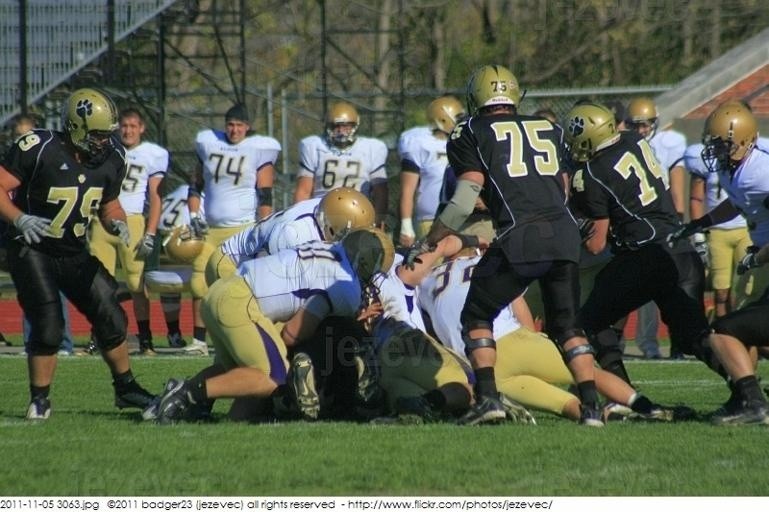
left=138, top=333, right=210, bottom=358
left=19, top=341, right=103, bottom=358
left=351, top=341, right=381, bottom=405
left=288, top=350, right=322, bottom=421
left=111, top=374, right=214, bottom=427
left=701, top=374, right=769, bottom=426
left=453, top=390, right=508, bottom=427
left=394, top=394, right=437, bottom=426
left=23, top=396, right=53, bottom=421
left=577, top=402, right=698, bottom=428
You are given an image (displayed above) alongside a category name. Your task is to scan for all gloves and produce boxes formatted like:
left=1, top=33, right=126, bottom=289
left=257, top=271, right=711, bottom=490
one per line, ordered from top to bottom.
left=666, top=211, right=714, bottom=251
left=16, top=214, right=54, bottom=245
left=573, top=215, right=598, bottom=246
left=190, top=216, right=209, bottom=239
left=111, top=217, right=131, bottom=248
left=692, top=232, right=712, bottom=268
left=134, top=234, right=157, bottom=261
left=402, top=235, right=437, bottom=271
left=734, top=245, right=762, bottom=277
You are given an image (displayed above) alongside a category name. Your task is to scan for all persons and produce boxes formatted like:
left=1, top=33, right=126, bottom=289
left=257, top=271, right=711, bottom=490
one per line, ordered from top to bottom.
left=1, top=64, right=769, bottom=428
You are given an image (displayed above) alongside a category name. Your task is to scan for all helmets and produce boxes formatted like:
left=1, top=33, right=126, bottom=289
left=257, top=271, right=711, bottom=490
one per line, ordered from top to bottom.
left=467, top=62, right=521, bottom=112
left=60, top=86, right=121, bottom=158
left=426, top=95, right=467, bottom=136
left=624, top=95, right=658, bottom=126
left=703, top=97, right=759, bottom=164
left=561, top=100, right=622, bottom=162
left=322, top=101, right=362, bottom=134
left=161, top=223, right=206, bottom=266
